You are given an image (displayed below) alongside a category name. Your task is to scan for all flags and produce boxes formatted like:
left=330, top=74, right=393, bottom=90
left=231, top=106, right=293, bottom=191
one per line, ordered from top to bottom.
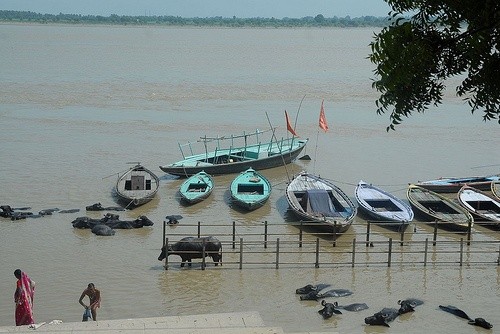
left=319, top=99, right=328, bottom=131
left=285, top=112, right=297, bottom=135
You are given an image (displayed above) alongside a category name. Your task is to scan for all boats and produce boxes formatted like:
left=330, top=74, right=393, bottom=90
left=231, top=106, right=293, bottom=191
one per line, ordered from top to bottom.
left=159, top=137, right=310, bottom=177
left=115, top=162, right=160, bottom=206
left=229, top=166, right=272, bottom=212
left=285, top=169, right=357, bottom=235
left=178, top=170, right=216, bottom=205
left=491, top=177, right=500, bottom=200
left=354, top=179, right=414, bottom=231
left=407, top=182, right=474, bottom=231
left=456, top=183, right=500, bottom=230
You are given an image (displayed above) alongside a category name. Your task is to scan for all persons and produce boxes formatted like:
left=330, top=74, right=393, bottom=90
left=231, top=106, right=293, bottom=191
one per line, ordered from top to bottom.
left=13, top=269, right=35, bottom=326
left=79, top=283, right=101, bottom=321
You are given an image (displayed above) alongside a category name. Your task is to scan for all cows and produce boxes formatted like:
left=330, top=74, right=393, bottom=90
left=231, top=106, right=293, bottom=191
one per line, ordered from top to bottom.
left=85, top=203, right=131, bottom=211
left=0, top=205, right=41, bottom=221
left=39, top=208, right=80, bottom=216
left=71, top=213, right=154, bottom=236
left=166, top=215, right=183, bottom=225
left=158, top=236, right=222, bottom=268
left=296, top=284, right=352, bottom=301
left=398, top=298, right=423, bottom=313
left=318, top=299, right=368, bottom=318
left=439, top=305, right=493, bottom=329
left=364, top=308, right=400, bottom=328
